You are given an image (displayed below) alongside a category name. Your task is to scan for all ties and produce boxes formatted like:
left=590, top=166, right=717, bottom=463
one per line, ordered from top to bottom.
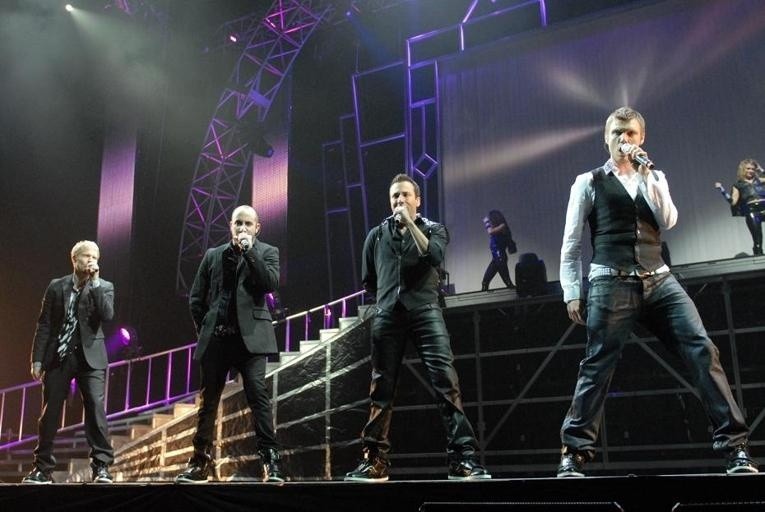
left=57, top=282, right=83, bottom=362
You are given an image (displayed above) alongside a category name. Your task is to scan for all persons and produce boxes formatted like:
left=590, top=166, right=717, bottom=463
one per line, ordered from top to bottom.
left=557, top=105, right=762, bottom=478
left=715, top=159, right=765, bottom=256
left=344, top=173, right=491, bottom=480
left=174, top=204, right=288, bottom=485
left=481, top=214, right=517, bottom=288
left=23, top=240, right=116, bottom=485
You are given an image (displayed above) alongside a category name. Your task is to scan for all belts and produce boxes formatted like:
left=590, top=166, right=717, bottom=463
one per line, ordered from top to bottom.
left=620, top=267, right=659, bottom=278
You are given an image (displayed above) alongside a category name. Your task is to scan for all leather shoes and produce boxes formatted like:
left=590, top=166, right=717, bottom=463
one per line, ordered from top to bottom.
left=557, top=447, right=585, bottom=479
left=727, top=447, right=759, bottom=476
left=448, top=458, right=492, bottom=478
left=344, top=459, right=390, bottom=483
left=262, top=461, right=291, bottom=482
left=174, top=464, right=208, bottom=483
left=22, top=466, right=52, bottom=485
left=91, top=466, right=112, bottom=483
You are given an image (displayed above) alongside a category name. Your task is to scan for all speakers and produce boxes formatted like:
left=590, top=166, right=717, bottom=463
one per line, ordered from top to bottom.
left=515, top=260, right=547, bottom=295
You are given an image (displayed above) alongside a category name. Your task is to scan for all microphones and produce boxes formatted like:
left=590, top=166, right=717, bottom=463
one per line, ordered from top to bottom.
left=621, top=143, right=654, bottom=169
left=394, top=214, right=402, bottom=224
left=240, top=238, right=249, bottom=250
left=89, top=266, right=100, bottom=273
